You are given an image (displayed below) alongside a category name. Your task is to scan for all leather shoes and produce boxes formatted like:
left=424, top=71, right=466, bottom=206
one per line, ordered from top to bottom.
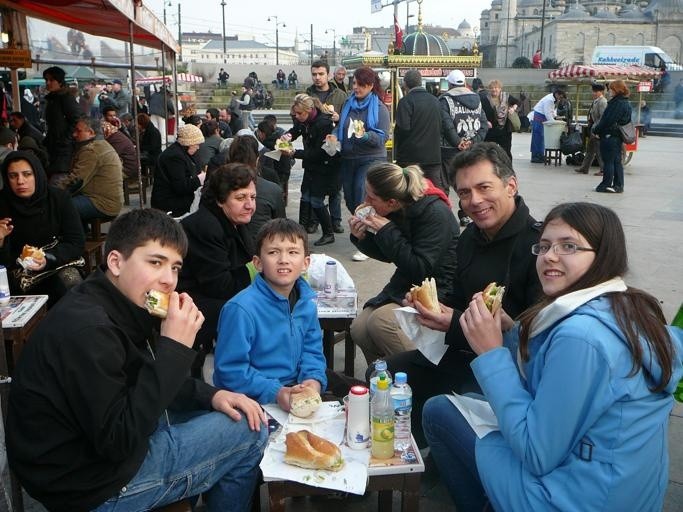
left=531, top=157, right=544, bottom=163
left=575, top=168, right=588, bottom=174
left=595, top=171, right=604, bottom=176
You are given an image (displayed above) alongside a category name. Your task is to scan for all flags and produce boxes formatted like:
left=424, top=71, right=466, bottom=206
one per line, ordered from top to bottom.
left=394, top=16, right=402, bottom=48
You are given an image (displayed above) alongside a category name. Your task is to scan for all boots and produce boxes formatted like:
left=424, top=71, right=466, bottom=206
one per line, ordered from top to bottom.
left=299, top=197, right=310, bottom=230
left=307, top=206, right=319, bottom=232
left=313, top=207, right=335, bottom=246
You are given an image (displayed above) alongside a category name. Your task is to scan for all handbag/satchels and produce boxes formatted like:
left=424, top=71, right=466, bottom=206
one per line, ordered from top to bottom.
left=507, top=111, right=521, bottom=132
left=620, top=122, right=636, bottom=144
left=11, top=236, right=86, bottom=290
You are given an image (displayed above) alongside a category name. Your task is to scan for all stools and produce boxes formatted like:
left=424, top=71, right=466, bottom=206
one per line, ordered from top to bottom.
left=545, top=149, right=561, bottom=167
left=145, top=164, right=154, bottom=187
left=123, top=176, right=146, bottom=206
left=86, top=216, right=116, bottom=241
left=84, top=242, right=104, bottom=274
left=317, top=287, right=358, bottom=379
left=0, top=295, right=48, bottom=377
left=268, top=398, right=426, bottom=512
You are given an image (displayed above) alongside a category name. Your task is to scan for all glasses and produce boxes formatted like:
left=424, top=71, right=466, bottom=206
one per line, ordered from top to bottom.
left=530, top=242, right=594, bottom=255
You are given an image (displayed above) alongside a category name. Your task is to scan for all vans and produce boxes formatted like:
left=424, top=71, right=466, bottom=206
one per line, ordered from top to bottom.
left=592, top=46, right=683, bottom=73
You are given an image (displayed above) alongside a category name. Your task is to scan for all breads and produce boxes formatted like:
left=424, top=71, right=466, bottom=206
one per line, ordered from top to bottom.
left=288, top=384, right=322, bottom=418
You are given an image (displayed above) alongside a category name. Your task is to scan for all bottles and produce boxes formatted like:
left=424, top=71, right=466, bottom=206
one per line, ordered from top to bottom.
left=324, top=260, right=337, bottom=298
left=0, top=264, right=11, bottom=306
left=369, top=359, right=393, bottom=402
left=390, top=372, right=412, bottom=451
left=370, top=380, right=396, bottom=459
left=346, top=385, right=370, bottom=450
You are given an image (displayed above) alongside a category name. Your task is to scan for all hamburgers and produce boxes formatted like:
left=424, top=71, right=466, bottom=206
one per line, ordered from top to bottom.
left=323, top=103, right=336, bottom=115
left=353, top=119, right=360, bottom=133
left=277, top=139, right=292, bottom=151
left=354, top=202, right=369, bottom=215
left=22, top=244, right=47, bottom=264
left=410, top=276, right=442, bottom=314
left=482, top=282, right=506, bottom=312
left=144, top=289, right=183, bottom=317
left=283, top=430, right=344, bottom=472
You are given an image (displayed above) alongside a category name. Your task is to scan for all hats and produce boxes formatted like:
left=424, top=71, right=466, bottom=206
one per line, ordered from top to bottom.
left=445, top=69, right=465, bottom=85
left=113, top=79, right=121, bottom=84
left=591, top=81, right=604, bottom=89
left=177, top=123, right=205, bottom=146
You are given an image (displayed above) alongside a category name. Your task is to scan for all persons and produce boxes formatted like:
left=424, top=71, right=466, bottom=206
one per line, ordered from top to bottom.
left=532, top=50, right=543, bottom=69
left=0, top=63, right=683, bottom=342
left=366, top=143, right=544, bottom=445
left=348, top=163, right=462, bottom=366
left=421, top=202, right=682, bottom=511
left=3, top=209, right=269, bottom=512
left=213, top=218, right=368, bottom=404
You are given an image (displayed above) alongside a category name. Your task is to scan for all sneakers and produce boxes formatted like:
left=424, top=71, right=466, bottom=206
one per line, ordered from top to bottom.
left=606, top=185, right=623, bottom=192
left=460, top=216, right=473, bottom=226
left=333, top=224, right=344, bottom=233
left=352, top=250, right=369, bottom=261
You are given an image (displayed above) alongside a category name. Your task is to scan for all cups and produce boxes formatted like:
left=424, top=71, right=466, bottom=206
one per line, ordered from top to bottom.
left=343, top=395, right=350, bottom=426
